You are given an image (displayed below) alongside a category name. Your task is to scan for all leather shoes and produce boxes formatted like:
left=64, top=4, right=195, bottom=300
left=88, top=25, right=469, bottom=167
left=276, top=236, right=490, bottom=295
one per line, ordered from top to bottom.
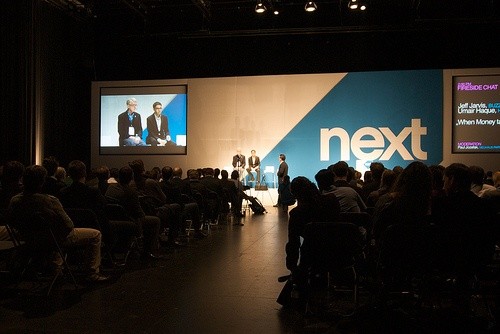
left=82, top=270, right=113, bottom=287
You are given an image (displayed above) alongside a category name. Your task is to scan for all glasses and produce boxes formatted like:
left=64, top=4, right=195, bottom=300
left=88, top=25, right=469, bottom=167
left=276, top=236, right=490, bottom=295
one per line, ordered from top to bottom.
left=128, top=103, right=137, bottom=107
left=155, top=107, right=163, bottom=110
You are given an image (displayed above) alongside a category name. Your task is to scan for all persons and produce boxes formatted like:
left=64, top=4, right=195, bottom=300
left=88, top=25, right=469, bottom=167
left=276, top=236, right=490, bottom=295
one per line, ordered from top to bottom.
left=118, top=100, right=142, bottom=147
left=145, top=102, right=176, bottom=146
left=232, top=148, right=248, bottom=182
left=246, top=150, right=261, bottom=183
left=272, top=154, right=288, bottom=207
left=41, top=159, right=67, bottom=204
left=84, top=159, right=245, bottom=239
left=61, top=160, right=127, bottom=268
left=315, top=160, right=500, bottom=273
left=0, top=161, right=27, bottom=241
left=7, top=165, right=114, bottom=286
left=54, top=166, right=68, bottom=190
left=103, top=167, right=164, bottom=259
left=278, top=176, right=295, bottom=213
left=285, top=176, right=349, bottom=276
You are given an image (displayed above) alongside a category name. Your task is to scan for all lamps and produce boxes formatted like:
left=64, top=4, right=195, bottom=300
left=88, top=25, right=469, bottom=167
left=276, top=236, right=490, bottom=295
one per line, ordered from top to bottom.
left=305, top=0, right=317, bottom=12
left=348, top=0, right=359, bottom=10
left=255, top=1, right=267, bottom=14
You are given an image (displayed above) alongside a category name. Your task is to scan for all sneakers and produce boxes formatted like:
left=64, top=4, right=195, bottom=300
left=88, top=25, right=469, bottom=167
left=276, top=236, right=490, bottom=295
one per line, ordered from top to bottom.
left=273, top=203, right=278, bottom=207
left=111, top=211, right=247, bottom=260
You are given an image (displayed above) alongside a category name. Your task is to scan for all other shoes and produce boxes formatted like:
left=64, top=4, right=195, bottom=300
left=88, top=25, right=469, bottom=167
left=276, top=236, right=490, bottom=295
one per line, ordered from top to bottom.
left=250, top=175, right=260, bottom=183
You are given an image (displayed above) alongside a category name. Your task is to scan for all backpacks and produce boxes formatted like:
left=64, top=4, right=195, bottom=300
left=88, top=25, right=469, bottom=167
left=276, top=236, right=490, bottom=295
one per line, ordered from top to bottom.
left=249, top=197, right=265, bottom=215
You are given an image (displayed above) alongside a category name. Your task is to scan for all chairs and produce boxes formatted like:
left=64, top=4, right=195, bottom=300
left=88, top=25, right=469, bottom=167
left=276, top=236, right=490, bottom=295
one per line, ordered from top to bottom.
left=1, top=189, right=239, bottom=293
left=304, top=201, right=500, bottom=318
